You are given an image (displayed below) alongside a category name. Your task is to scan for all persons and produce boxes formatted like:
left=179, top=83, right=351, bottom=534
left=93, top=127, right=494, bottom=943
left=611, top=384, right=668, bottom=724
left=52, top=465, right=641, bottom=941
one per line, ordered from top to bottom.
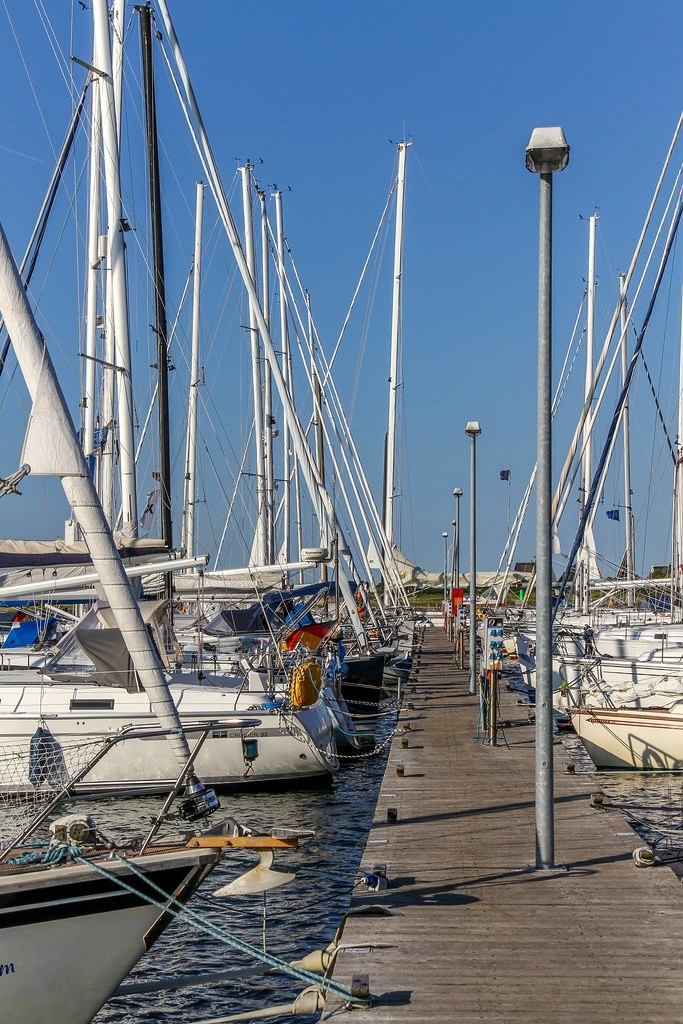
left=583, top=624, right=594, bottom=659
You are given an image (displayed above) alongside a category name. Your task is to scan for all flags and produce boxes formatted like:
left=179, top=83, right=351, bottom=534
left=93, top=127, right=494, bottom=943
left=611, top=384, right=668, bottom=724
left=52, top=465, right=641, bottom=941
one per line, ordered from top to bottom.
left=498, top=467, right=509, bottom=482
left=606, top=510, right=619, bottom=521
left=355, top=587, right=369, bottom=621
left=11, top=610, right=29, bottom=625
left=283, top=619, right=337, bottom=651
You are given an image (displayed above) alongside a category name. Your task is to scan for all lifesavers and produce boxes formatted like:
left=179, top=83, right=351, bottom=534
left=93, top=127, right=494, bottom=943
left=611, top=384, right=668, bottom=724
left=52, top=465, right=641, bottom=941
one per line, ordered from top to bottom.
left=291, top=662, right=321, bottom=708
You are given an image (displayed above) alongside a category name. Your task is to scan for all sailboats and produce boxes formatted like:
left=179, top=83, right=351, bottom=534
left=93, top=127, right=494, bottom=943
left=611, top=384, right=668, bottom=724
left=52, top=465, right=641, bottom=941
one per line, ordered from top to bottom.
left=0, top=0, right=417, bottom=794
left=501, top=112, right=683, bottom=773
left=1, top=217, right=296, bottom=1024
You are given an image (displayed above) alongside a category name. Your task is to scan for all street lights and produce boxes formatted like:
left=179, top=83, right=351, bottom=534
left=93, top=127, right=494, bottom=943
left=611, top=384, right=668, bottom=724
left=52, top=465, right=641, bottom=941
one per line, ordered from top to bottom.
left=524, top=127, right=569, bottom=868
left=465, top=421, right=482, bottom=696
left=453, top=488, right=464, bottom=588
left=450, top=520, right=456, bottom=599
left=442, top=532, right=448, bottom=601
